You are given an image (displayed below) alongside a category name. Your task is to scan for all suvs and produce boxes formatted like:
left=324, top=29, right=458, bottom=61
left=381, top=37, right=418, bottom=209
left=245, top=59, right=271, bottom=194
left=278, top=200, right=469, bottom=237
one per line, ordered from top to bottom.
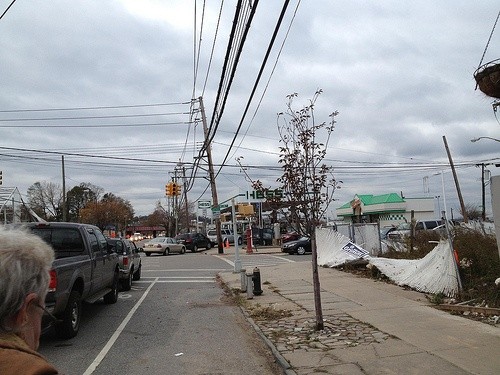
left=242, top=228, right=273, bottom=246
left=207, top=229, right=242, bottom=248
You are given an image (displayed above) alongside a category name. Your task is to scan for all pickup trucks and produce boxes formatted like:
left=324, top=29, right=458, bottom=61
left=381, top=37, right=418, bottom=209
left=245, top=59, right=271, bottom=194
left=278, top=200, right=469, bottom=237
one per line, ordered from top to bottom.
left=7, top=222, right=119, bottom=340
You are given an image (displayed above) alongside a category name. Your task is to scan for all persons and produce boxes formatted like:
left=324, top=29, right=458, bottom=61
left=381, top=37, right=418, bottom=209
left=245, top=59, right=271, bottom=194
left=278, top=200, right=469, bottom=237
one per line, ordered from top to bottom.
left=0, top=223, right=60, bottom=375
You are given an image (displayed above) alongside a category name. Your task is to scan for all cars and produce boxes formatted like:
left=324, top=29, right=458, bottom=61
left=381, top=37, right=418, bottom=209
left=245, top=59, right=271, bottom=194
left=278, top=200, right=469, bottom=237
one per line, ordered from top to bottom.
left=282, top=231, right=299, bottom=243
left=174, top=233, right=212, bottom=253
left=281, top=235, right=311, bottom=255
left=104, top=236, right=142, bottom=291
left=142, top=237, right=186, bottom=256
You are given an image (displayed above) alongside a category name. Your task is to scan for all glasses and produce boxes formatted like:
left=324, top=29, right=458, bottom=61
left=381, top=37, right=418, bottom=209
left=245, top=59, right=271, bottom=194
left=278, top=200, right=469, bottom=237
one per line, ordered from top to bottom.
left=32, top=300, right=58, bottom=336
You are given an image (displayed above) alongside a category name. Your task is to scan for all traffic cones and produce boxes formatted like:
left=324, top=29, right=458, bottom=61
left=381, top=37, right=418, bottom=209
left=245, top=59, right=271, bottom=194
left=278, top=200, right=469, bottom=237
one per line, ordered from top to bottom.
left=226, top=238, right=229, bottom=249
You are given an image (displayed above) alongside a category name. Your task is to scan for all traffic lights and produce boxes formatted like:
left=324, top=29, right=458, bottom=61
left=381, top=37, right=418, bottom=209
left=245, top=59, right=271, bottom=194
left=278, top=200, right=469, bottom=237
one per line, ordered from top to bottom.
left=165, top=183, right=171, bottom=196
left=172, top=183, right=178, bottom=196
left=177, top=184, right=182, bottom=195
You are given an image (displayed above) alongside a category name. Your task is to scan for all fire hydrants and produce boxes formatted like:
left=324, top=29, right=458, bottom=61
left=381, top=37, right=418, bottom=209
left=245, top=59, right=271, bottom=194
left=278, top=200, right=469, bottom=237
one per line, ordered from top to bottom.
left=251, top=267, right=264, bottom=296
